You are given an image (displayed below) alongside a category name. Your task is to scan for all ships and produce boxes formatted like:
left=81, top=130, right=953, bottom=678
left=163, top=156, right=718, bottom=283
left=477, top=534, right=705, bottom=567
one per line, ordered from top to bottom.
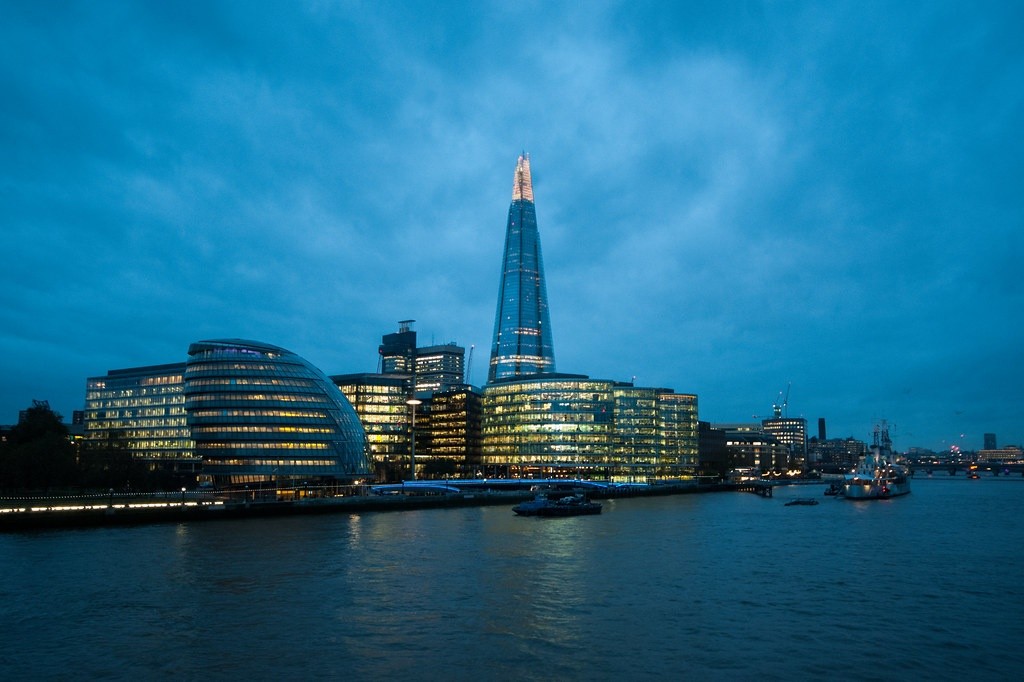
left=842, top=425, right=911, bottom=500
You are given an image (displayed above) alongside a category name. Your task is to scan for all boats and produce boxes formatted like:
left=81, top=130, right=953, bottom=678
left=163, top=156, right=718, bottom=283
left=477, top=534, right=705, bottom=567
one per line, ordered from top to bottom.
left=510, top=498, right=603, bottom=518
